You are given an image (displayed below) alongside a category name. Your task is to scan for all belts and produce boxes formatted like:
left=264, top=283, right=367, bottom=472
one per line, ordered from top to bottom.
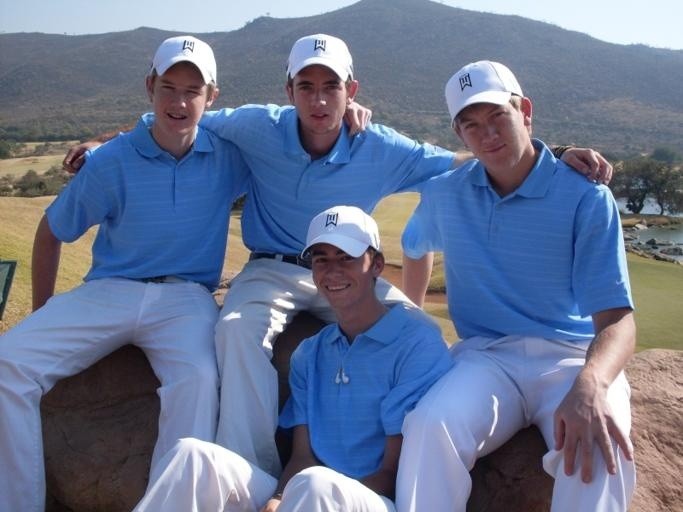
left=249, top=251, right=312, bottom=270
left=142, top=276, right=187, bottom=283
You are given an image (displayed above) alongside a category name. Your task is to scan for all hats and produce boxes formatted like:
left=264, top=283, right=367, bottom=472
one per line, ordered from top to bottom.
left=285, top=33, right=353, bottom=83
left=148, top=35, right=218, bottom=88
left=443, top=60, right=524, bottom=126
left=301, top=205, right=380, bottom=259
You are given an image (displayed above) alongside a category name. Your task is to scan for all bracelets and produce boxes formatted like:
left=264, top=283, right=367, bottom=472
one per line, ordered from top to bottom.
left=552, top=143, right=574, bottom=162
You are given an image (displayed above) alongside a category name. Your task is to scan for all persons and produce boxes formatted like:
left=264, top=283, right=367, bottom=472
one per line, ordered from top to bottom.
left=1, top=33, right=253, bottom=511
left=59, top=33, right=615, bottom=480
left=393, top=59, right=641, bottom=512
left=132, top=201, right=458, bottom=512
